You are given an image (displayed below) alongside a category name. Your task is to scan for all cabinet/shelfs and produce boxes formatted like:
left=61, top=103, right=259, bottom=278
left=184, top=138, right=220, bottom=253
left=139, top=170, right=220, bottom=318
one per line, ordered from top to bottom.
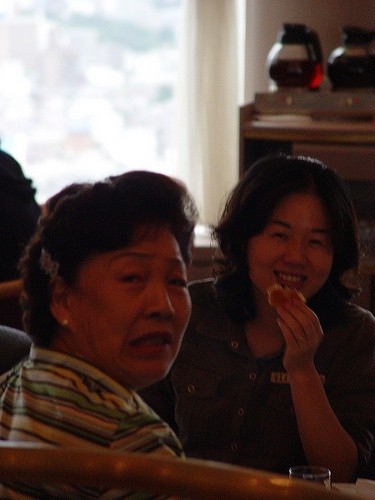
left=238, top=87, right=375, bottom=312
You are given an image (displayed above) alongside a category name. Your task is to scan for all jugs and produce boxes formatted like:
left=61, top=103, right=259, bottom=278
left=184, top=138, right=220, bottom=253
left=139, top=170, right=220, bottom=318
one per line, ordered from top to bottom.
left=266, top=23, right=324, bottom=89
left=325, top=25, right=375, bottom=90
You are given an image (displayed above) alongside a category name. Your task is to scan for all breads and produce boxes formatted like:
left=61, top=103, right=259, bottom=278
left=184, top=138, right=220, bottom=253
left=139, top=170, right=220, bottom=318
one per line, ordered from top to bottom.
left=267, top=284, right=307, bottom=308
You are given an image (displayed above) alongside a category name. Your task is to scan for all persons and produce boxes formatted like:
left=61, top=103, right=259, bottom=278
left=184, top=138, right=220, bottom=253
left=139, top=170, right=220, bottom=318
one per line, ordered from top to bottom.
left=0, top=148, right=43, bottom=283
left=138, top=153, right=375, bottom=486
left=0, top=168, right=201, bottom=460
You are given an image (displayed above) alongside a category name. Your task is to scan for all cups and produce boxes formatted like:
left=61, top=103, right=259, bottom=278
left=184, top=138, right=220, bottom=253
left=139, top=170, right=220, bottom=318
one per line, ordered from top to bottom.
left=289, top=466, right=331, bottom=491
